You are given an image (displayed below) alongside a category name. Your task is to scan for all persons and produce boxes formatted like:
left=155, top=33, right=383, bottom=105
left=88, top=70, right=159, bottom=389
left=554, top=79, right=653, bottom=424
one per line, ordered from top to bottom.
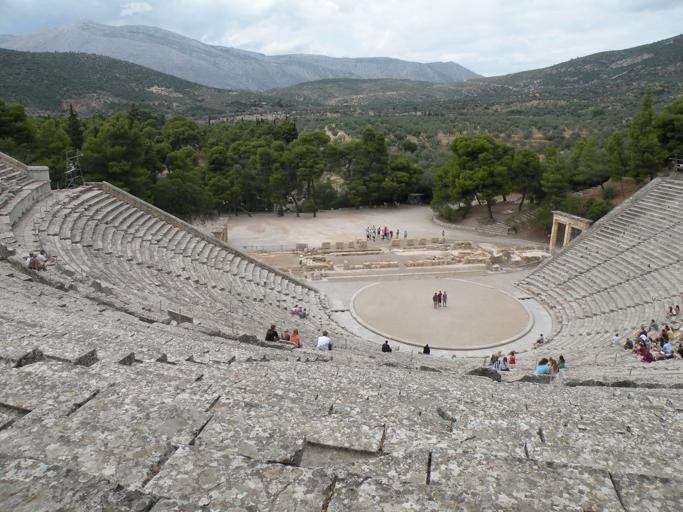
left=366, top=224, right=407, bottom=242
left=433, top=291, right=447, bottom=309
left=624, top=304, right=683, bottom=363
left=292, top=305, right=309, bottom=319
left=265, top=324, right=280, bottom=342
left=289, top=328, right=302, bottom=347
left=281, top=329, right=289, bottom=341
left=318, top=331, right=332, bottom=350
left=537, top=333, right=544, bottom=343
left=610, top=333, right=620, bottom=347
left=382, top=340, right=391, bottom=353
left=424, top=344, right=431, bottom=354
left=491, top=351, right=516, bottom=371
left=536, top=354, right=566, bottom=375
